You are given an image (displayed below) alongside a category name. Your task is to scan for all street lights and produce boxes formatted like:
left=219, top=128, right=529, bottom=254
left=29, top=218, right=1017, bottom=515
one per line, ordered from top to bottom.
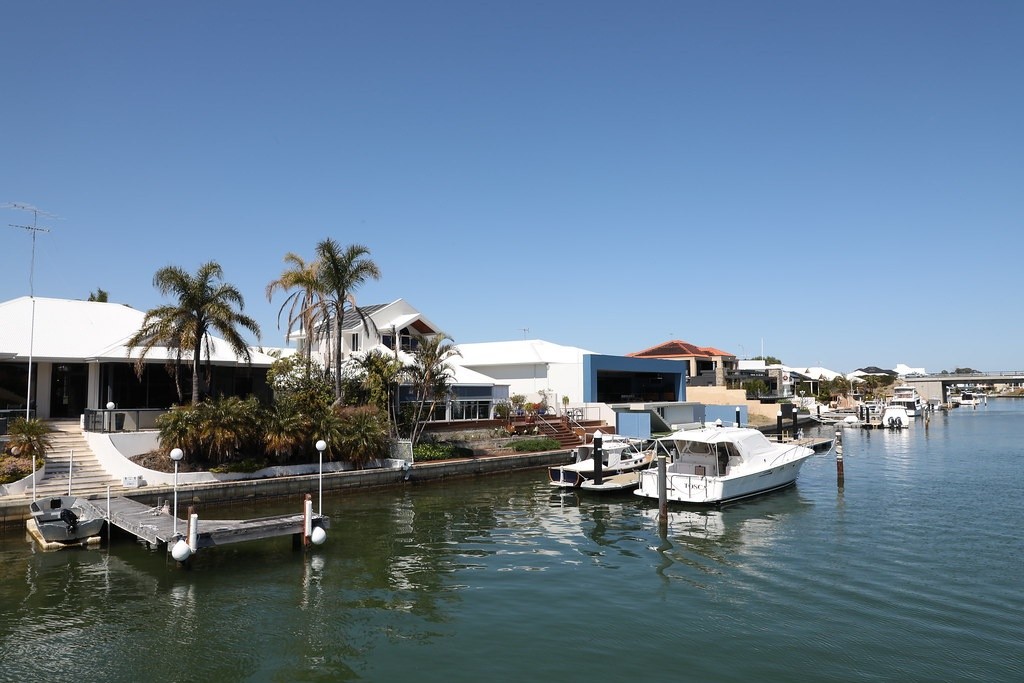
left=316, top=440, right=326, bottom=516
left=170, top=449, right=183, bottom=533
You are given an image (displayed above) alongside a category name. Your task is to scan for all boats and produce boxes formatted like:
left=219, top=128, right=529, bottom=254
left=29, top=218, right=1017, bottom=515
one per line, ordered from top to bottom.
left=857, top=385, right=928, bottom=420
left=951, top=391, right=986, bottom=405
left=633, top=419, right=815, bottom=502
left=548, top=430, right=673, bottom=489
left=30, top=493, right=104, bottom=540
